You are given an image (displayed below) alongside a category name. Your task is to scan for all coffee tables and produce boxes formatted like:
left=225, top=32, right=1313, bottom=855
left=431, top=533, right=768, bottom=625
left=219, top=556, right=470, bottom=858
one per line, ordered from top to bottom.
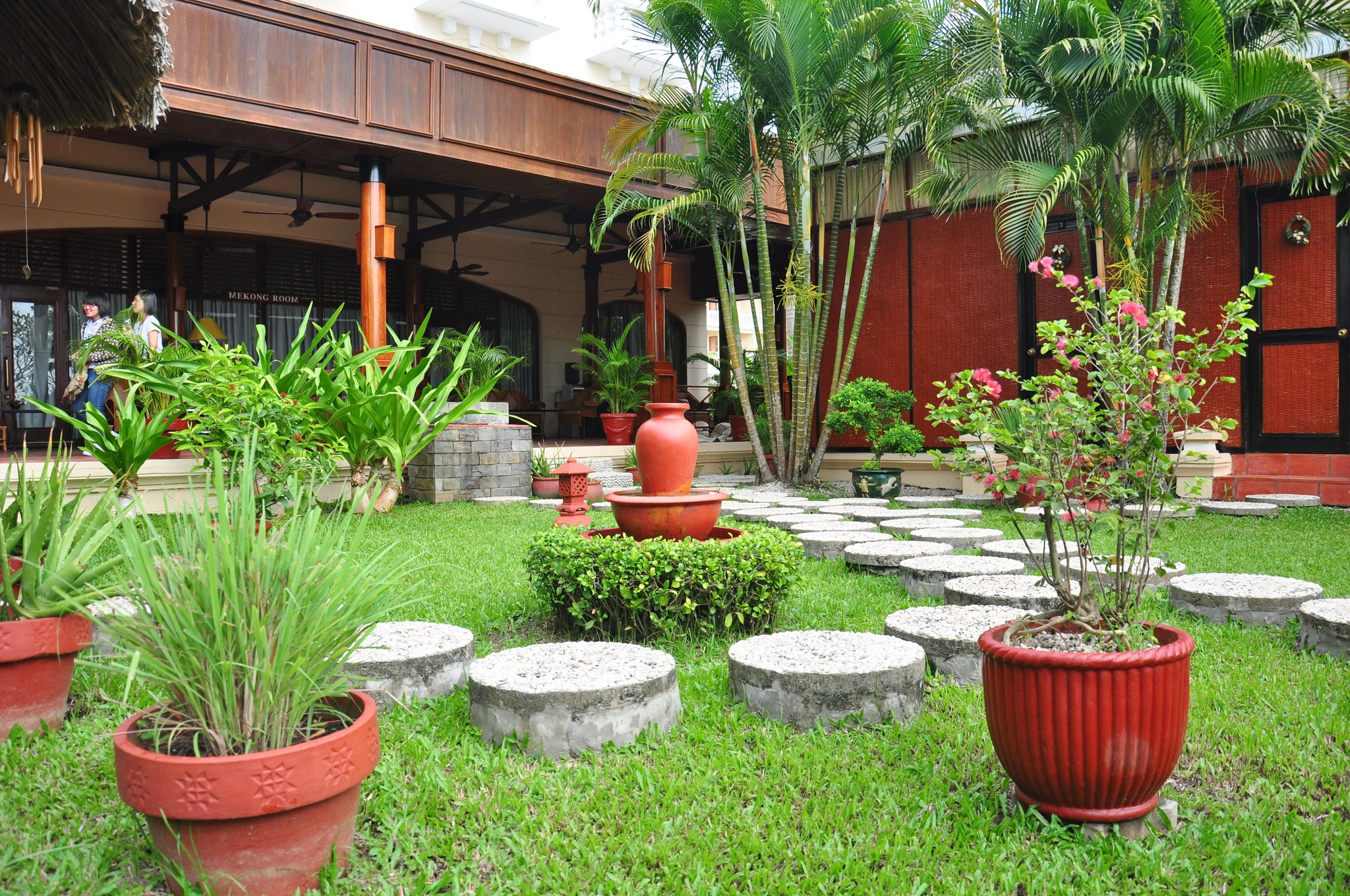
left=0, top=407, right=76, bottom=452
left=508, top=408, right=564, bottom=441
left=685, top=408, right=717, bottom=437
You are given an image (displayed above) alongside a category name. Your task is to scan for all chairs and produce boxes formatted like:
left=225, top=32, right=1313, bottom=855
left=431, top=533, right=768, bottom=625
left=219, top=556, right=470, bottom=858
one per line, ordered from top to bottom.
left=100, top=376, right=136, bottom=434
left=552, top=385, right=606, bottom=440
left=677, top=389, right=714, bottom=431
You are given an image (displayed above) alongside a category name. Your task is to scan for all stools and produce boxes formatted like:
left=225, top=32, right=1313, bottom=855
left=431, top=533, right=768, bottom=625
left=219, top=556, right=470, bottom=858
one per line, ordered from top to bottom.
left=0, top=426, right=8, bottom=452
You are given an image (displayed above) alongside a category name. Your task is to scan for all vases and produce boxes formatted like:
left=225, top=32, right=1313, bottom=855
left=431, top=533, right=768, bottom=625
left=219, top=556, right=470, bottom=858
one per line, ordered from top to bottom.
left=570, top=313, right=659, bottom=446
left=10, top=405, right=21, bottom=410
left=975, top=614, right=1197, bottom=822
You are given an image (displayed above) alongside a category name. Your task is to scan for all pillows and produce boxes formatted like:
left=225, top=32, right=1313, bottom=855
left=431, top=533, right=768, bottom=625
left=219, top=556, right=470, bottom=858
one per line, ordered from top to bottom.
left=107, top=383, right=129, bottom=411
left=487, top=392, right=497, bottom=402
left=504, top=393, right=518, bottom=410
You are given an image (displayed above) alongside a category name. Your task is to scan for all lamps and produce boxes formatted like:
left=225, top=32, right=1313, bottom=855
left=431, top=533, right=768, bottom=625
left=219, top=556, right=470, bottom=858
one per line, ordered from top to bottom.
left=188, top=317, right=229, bottom=350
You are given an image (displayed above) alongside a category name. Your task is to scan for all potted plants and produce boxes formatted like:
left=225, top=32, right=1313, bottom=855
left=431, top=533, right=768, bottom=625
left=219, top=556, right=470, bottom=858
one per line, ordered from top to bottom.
left=63, top=290, right=211, bottom=460
left=164, top=341, right=353, bottom=543
left=682, top=345, right=793, bottom=442
left=821, top=376, right=927, bottom=500
left=6, top=401, right=478, bottom=896
left=738, top=411, right=792, bottom=476
left=0, top=417, right=162, bottom=745
left=528, top=438, right=573, bottom=500
left=616, top=442, right=641, bottom=485
left=581, top=456, right=603, bottom=504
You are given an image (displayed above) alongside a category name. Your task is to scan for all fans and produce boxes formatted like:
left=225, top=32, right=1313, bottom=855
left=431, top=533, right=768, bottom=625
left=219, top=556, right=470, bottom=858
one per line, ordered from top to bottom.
left=240, top=159, right=360, bottom=228
left=530, top=221, right=615, bottom=255
left=426, top=231, right=490, bottom=278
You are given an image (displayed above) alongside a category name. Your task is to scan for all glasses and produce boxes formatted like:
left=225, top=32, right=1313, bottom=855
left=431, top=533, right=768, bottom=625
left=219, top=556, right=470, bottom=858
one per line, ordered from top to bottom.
left=82, top=302, right=97, bottom=308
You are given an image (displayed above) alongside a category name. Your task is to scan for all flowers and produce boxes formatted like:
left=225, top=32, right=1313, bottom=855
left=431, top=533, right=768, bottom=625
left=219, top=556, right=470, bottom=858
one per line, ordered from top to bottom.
left=922, top=253, right=1275, bottom=648
left=5, top=395, right=25, bottom=406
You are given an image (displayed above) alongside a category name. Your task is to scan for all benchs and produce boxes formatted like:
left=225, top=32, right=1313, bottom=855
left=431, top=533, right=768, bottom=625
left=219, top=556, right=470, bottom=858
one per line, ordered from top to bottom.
left=485, top=388, right=546, bottom=440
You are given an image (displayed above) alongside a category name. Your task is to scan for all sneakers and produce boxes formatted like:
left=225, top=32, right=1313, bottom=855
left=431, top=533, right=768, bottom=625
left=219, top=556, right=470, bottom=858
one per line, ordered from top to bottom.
left=110, top=431, right=119, bottom=438
left=83, top=443, right=105, bottom=456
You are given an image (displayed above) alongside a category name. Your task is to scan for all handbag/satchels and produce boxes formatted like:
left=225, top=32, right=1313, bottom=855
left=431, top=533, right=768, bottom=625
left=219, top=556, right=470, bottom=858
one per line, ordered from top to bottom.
left=61, top=361, right=89, bottom=406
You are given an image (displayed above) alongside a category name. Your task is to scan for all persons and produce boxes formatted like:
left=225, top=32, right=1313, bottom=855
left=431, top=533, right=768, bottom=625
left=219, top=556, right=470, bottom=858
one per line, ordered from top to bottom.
left=123, top=289, right=164, bottom=419
left=70, top=291, right=123, bottom=457
